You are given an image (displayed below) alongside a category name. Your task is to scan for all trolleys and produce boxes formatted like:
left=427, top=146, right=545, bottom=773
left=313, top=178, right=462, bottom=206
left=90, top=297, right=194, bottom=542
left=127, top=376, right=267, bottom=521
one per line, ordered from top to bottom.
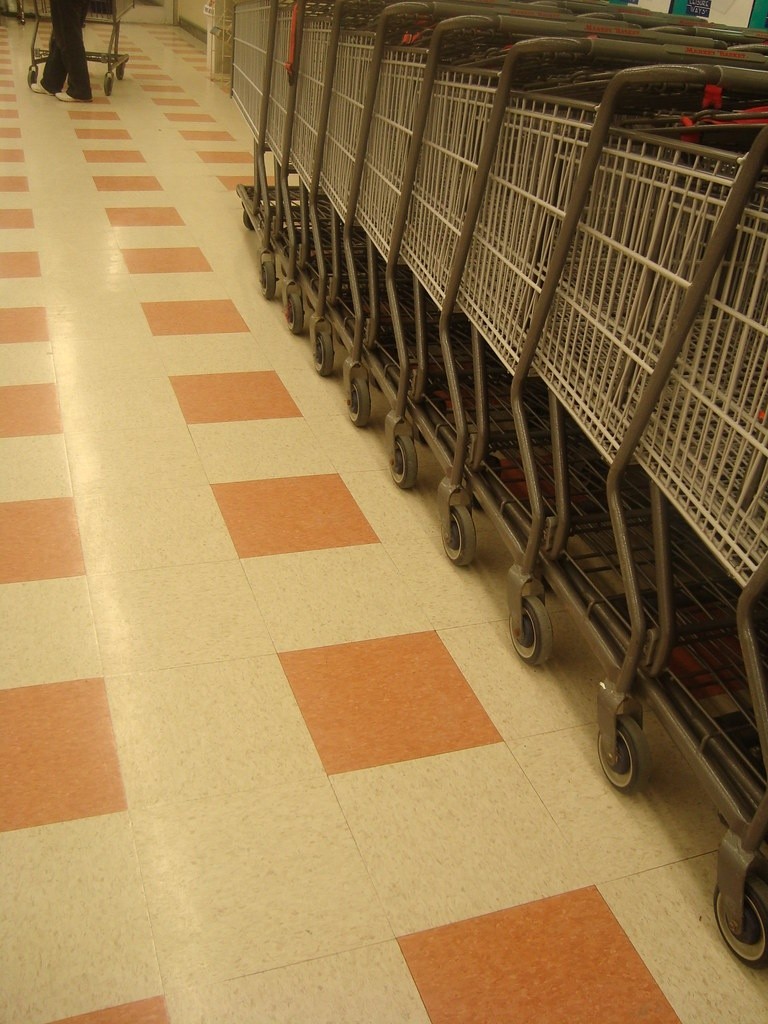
left=26, top=0, right=135, bottom=95
left=213, top=1, right=767, bottom=981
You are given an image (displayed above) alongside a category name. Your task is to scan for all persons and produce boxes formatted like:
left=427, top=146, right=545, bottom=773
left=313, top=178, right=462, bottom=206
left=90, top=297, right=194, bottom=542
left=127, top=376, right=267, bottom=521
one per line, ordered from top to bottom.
left=30, top=0, right=93, bottom=102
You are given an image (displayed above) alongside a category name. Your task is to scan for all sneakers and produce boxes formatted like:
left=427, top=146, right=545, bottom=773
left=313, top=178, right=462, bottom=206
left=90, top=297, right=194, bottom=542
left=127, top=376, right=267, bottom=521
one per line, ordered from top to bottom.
left=31, top=82, right=56, bottom=96
left=55, top=91, right=94, bottom=102
